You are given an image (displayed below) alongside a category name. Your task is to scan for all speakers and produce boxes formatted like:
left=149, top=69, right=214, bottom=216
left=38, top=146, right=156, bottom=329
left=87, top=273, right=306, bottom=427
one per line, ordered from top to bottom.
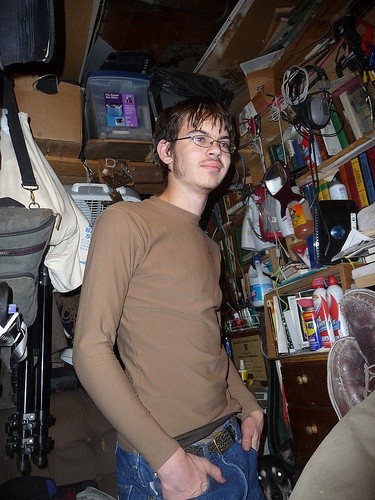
left=315, top=200, right=357, bottom=266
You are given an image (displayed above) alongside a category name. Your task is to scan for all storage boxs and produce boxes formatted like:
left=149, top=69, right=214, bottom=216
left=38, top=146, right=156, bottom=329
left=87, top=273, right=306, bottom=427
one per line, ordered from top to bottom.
left=240, top=65, right=276, bottom=111
left=81, top=69, right=154, bottom=142
left=13, top=74, right=83, bottom=157
left=230, top=334, right=268, bottom=385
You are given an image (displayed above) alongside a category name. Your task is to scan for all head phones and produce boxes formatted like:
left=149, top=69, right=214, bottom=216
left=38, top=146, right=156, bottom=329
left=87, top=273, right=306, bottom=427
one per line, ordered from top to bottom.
left=288, top=65, right=331, bottom=131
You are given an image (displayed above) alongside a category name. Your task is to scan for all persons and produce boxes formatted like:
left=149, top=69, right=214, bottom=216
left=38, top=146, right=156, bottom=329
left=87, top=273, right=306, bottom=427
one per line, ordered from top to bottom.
left=73, top=96, right=265, bottom=500
left=286, top=288, right=375, bottom=499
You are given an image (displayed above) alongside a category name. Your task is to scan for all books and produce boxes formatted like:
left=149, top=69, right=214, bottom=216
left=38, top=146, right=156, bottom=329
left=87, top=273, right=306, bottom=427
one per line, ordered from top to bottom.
left=261, top=6, right=299, bottom=54
left=267, top=78, right=375, bottom=179
left=303, top=147, right=375, bottom=210
left=351, top=246, right=375, bottom=289
left=267, top=289, right=313, bottom=354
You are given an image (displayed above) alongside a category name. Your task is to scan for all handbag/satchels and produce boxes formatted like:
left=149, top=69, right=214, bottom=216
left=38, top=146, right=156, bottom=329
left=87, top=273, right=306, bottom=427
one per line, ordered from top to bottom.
left=0, top=202, right=61, bottom=327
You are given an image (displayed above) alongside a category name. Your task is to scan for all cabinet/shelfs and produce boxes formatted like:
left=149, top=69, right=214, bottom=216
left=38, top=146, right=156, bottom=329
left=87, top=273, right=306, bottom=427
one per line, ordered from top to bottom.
left=197, top=0, right=375, bottom=375
left=278, top=351, right=340, bottom=477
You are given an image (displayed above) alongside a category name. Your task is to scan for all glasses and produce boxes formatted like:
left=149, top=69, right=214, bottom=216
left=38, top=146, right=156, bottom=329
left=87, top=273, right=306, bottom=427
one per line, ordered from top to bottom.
left=166, top=133, right=236, bottom=154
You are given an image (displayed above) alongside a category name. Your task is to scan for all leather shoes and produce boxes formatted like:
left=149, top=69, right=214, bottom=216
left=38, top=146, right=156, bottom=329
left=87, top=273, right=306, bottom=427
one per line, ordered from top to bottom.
left=339, top=288, right=375, bottom=384
left=326, top=335, right=375, bottom=420
